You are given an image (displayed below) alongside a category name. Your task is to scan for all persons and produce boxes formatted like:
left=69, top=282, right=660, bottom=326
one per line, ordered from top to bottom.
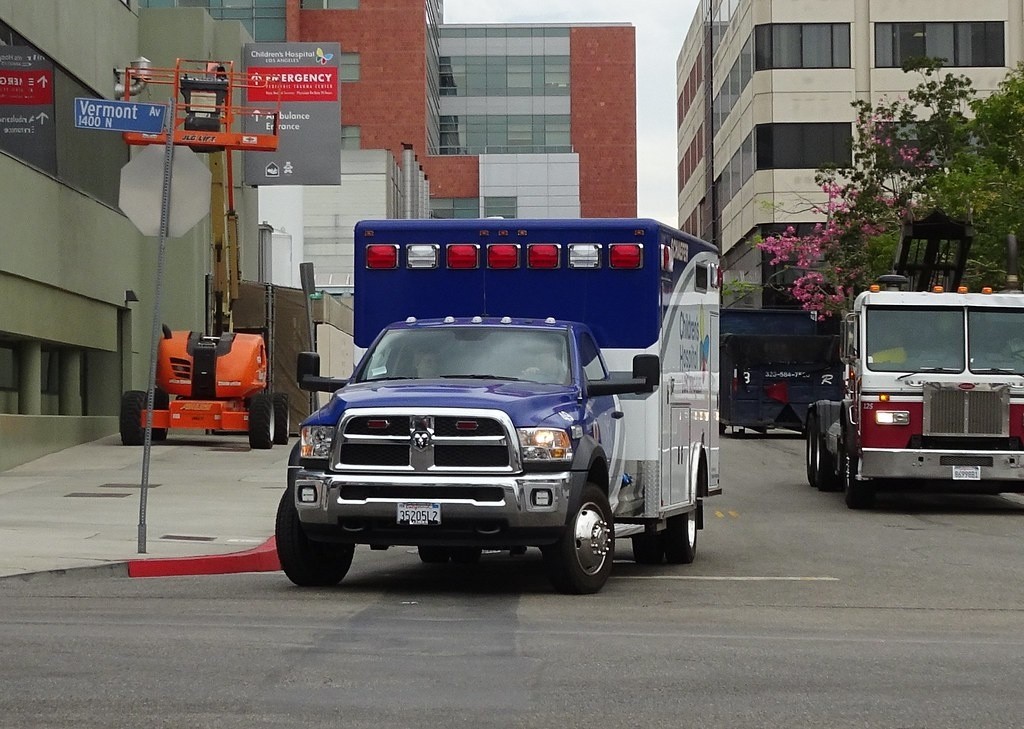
left=520, top=346, right=571, bottom=384
left=414, top=347, right=442, bottom=378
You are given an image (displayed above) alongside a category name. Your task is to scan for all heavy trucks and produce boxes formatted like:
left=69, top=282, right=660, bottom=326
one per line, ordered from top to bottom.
left=805, top=275, right=1024, bottom=513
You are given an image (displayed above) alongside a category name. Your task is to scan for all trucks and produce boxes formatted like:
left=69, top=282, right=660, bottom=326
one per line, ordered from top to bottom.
left=274, top=217, right=721, bottom=598
left=716, top=306, right=846, bottom=436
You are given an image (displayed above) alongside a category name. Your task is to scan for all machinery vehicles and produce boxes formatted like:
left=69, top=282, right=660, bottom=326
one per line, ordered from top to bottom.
left=119, top=58, right=291, bottom=451
